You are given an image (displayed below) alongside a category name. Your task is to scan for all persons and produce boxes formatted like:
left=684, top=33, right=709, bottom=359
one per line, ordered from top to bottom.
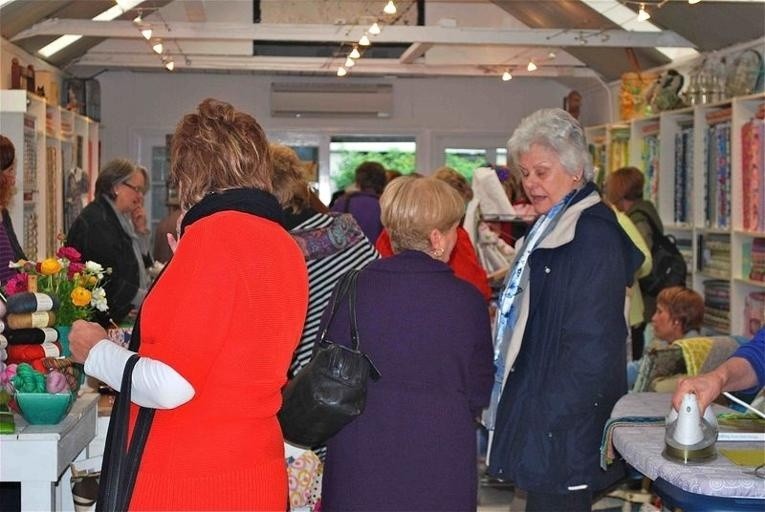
left=2, top=95, right=765, bottom=512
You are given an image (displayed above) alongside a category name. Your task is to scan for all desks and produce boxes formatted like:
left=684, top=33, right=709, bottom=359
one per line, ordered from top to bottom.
left=611, top=390, right=765, bottom=512
left=0, top=392, right=98, bottom=512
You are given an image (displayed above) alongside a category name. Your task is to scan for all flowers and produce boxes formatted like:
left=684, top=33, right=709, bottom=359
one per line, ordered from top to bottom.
left=8, top=233, right=112, bottom=325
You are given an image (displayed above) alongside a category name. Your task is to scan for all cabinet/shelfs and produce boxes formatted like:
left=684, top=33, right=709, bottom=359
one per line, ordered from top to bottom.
left=0, top=89, right=99, bottom=260
left=585, top=94, right=765, bottom=337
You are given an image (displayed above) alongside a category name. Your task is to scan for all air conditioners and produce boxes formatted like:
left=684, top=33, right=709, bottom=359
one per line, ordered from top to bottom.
left=271, top=82, right=394, bottom=119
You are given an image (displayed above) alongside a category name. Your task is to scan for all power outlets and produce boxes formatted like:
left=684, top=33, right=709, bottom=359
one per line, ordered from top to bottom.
left=527, top=58, right=537, bottom=71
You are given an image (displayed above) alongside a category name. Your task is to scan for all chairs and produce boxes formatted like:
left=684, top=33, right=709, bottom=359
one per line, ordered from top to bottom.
left=631, top=337, right=748, bottom=393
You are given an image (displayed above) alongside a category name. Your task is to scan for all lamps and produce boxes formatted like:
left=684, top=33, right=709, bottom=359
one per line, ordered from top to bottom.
left=337, top=0, right=397, bottom=76
left=637, top=4, right=650, bottom=22
left=132, top=10, right=174, bottom=71
left=502, top=70, right=512, bottom=80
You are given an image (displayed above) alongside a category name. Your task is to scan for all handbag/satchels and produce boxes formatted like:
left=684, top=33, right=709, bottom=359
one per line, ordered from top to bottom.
left=275, top=269, right=381, bottom=451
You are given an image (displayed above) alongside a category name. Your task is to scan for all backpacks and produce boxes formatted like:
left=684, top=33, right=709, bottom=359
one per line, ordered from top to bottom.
left=626, top=208, right=687, bottom=298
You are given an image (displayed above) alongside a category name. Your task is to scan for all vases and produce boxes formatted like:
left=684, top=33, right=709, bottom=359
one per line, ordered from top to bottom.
left=54, top=325, right=71, bottom=358
